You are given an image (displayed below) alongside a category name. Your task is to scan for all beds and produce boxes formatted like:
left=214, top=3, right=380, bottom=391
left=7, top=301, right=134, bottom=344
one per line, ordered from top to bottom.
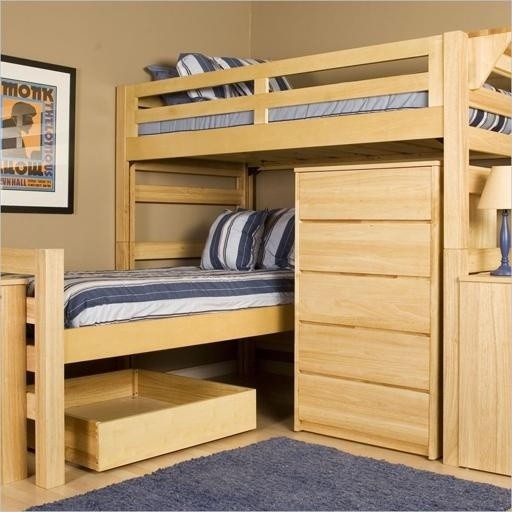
left=116, top=27, right=512, bottom=477
left=0, top=248, right=294, bottom=489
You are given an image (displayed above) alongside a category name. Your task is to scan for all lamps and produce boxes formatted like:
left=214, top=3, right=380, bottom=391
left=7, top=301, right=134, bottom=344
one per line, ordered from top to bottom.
left=478, top=166, right=512, bottom=276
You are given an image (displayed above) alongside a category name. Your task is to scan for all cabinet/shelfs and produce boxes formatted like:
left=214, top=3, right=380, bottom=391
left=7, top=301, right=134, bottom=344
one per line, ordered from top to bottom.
left=293, top=160, right=440, bottom=460
left=458, top=273, right=512, bottom=476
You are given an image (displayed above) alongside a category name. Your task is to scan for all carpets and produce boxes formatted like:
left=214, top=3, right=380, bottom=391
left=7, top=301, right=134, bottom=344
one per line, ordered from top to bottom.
left=25, top=436, right=512, bottom=512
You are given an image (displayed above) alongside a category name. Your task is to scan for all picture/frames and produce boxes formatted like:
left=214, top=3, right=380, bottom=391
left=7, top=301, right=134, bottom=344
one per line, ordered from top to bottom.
left=0, top=55, right=76, bottom=214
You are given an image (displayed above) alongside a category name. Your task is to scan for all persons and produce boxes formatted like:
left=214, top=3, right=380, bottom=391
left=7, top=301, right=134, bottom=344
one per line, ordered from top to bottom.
left=1, top=99, right=43, bottom=160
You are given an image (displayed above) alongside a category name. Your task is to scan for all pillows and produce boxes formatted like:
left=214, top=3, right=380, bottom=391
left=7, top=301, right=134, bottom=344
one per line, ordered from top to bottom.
left=146, top=53, right=292, bottom=105
left=258, top=208, right=295, bottom=269
left=199, top=209, right=269, bottom=271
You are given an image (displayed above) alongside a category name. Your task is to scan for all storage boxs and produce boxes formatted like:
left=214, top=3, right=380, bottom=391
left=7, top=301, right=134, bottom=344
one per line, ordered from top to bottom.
left=27, top=368, right=257, bottom=472
